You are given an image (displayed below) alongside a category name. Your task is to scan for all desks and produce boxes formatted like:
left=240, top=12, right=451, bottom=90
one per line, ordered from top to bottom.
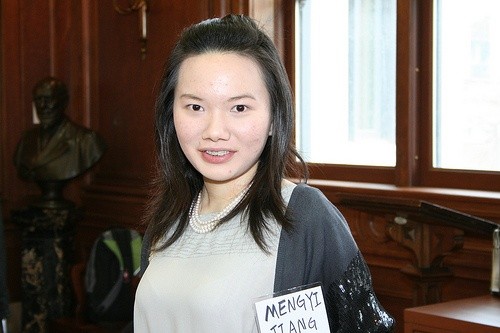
left=403, top=295, right=500, bottom=333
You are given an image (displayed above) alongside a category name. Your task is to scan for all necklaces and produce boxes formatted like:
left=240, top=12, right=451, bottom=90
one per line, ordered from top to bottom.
left=188, top=181, right=253, bottom=233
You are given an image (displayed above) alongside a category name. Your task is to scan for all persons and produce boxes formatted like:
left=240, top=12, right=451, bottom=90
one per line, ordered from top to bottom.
left=132, top=12, right=398, bottom=333
left=13, top=75, right=107, bottom=188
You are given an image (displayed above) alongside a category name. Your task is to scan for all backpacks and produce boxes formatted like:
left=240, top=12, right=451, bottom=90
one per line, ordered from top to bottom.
left=82, top=225, right=145, bottom=321
